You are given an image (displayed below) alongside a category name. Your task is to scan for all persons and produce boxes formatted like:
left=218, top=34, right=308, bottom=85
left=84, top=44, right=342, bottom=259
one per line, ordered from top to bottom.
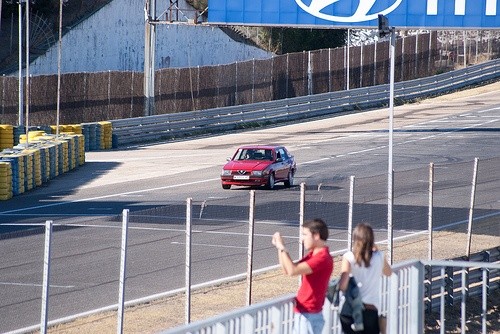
left=262, top=150, right=270, bottom=159
left=272, top=218, right=333, bottom=334
left=338, top=223, right=392, bottom=334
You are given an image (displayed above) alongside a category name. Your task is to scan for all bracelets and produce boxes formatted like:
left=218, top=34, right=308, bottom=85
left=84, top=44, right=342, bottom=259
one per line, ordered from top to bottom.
left=281, top=249, right=285, bottom=252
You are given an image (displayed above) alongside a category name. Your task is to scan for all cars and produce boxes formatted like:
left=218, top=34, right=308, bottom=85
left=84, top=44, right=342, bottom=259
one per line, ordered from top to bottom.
left=220, top=144, right=297, bottom=190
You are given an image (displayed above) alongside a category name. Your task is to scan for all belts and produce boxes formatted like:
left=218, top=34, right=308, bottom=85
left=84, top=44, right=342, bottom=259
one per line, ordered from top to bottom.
left=365, top=303, right=376, bottom=310
